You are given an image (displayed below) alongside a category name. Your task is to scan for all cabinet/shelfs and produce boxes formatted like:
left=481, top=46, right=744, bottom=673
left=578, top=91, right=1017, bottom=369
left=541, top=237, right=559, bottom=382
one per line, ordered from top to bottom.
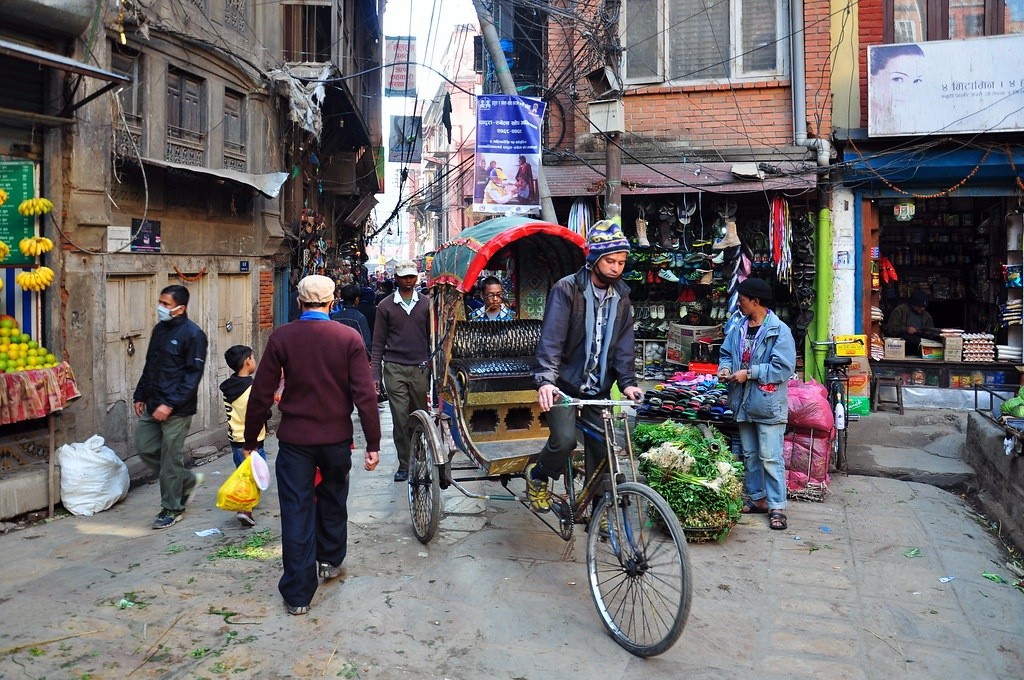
left=630, top=203, right=813, bottom=378
left=879, top=205, right=1022, bottom=363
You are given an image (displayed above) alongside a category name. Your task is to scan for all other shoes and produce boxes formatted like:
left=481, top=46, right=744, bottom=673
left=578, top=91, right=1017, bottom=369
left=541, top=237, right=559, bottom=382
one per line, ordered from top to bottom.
left=619, top=198, right=816, bottom=293
left=629, top=293, right=815, bottom=421
left=319, top=562, right=345, bottom=578
left=287, top=605, right=310, bottom=614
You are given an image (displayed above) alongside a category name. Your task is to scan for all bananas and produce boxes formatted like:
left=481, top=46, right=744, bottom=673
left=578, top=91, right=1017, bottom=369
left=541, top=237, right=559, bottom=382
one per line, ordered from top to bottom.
left=0, top=189, right=6, bottom=205
left=18, top=198, right=53, bottom=216
left=19, top=237, right=53, bottom=256
left=0, top=240, right=9, bottom=262
left=16, top=267, right=55, bottom=291
left=0, top=279, right=3, bottom=289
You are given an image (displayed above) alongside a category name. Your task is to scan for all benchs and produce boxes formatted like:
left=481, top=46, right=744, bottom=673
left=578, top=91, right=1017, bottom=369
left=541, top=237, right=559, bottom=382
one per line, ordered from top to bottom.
left=445, top=316, right=542, bottom=397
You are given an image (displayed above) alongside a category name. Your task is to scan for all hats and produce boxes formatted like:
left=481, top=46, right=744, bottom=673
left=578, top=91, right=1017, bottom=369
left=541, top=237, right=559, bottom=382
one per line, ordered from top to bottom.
left=490, top=168, right=507, bottom=179
left=586, top=220, right=630, bottom=265
left=395, top=260, right=419, bottom=276
left=298, top=275, right=335, bottom=303
left=737, top=278, right=773, bottom=306
left=911, top=292, right=928, bottom=306
left=687, top=298, right=708, bottom=315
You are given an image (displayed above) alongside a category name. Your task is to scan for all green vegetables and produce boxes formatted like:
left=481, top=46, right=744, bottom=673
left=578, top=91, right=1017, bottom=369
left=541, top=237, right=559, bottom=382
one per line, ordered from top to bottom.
left=630, top=418, right=745, bottom=544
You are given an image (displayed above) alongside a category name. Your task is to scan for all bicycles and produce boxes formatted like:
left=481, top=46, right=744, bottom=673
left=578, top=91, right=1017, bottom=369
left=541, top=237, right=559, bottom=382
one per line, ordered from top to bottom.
left=809, top=339, right=865, bottom=477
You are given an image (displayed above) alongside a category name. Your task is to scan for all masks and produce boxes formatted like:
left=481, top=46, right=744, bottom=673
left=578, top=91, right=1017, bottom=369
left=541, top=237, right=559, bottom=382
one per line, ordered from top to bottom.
left=156, top=304, right=183, bottom=321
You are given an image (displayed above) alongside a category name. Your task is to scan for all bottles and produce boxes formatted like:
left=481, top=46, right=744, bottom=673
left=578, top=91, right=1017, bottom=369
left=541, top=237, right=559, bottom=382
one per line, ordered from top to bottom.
left=943, top=230, right=962, bottom=242
left=883, top=231, right=901, bottom=241
left=906, top=232, right=911, bottom=242
left=929, top=232, right=942, bottom=242
left=912, top=244, right=933, bottom=266
left=957, top=244, right=971, bottom=268
left=934, top=245, right=956, bottom=268
left=890, top=246, right=910, bottom=266
left=898, top=278, right=918, bottom=299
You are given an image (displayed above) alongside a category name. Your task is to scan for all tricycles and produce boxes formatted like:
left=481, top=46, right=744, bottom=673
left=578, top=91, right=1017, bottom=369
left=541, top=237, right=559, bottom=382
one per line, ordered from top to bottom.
left=408, top=216, right=691, bottom=659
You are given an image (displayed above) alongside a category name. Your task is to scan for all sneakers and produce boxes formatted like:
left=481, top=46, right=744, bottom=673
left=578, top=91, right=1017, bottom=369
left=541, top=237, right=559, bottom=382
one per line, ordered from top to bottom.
left=525, top=463, right=551, bottom=513
left=181, top=474, right=201, bottom=507
left=152, top=508, right=183, bottom=529
left=237, top=511, right=255, bottom=525
left=591, top=511, right=619, bottom=536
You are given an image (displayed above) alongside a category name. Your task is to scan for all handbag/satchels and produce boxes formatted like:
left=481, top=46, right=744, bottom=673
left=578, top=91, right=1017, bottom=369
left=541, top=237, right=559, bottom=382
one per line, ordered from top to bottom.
left=376, top=381, right=387, bottom=402
left=216, top=454, right=261, bottom=511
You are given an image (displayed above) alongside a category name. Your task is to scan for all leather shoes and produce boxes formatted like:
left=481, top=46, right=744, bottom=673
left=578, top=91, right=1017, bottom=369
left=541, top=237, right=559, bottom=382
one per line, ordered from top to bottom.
left=394, top=471, right=409, bottom=481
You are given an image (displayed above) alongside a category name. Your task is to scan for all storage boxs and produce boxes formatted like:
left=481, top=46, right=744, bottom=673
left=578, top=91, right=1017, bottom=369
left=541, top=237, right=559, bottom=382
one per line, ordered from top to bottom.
left=831, top=335, right=872, bottom=417
left=919, top=337, right=944, bottom=360
left=943, top=337, right=963, bottom=351
left=884, top=339, right=905, bottom=359
left=944, top=350, right=961, bottom=362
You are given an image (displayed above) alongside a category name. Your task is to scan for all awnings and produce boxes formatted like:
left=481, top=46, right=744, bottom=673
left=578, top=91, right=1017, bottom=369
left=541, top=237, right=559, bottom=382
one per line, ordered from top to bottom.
left=0, top=38, right=130, bottom=115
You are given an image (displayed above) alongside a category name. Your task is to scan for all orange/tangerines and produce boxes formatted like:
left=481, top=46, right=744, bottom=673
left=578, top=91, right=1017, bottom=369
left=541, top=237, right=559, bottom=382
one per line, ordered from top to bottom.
left=0, top=319, right=60, bottom=374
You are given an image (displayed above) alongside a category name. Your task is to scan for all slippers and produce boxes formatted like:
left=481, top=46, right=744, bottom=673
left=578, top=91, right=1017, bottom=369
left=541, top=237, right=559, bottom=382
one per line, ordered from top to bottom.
left=740, top=500, right=769, bottom=513
left=769, top=512, right=787, bottom=529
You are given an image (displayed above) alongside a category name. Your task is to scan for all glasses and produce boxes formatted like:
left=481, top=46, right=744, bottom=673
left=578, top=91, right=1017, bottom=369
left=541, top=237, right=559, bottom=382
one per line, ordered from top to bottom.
left=484, top=291, right=506, bottom=299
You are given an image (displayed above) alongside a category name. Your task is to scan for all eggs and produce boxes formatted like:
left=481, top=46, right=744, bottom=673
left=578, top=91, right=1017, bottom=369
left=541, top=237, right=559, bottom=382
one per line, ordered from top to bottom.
left=965, top=339, right=993, bottom=360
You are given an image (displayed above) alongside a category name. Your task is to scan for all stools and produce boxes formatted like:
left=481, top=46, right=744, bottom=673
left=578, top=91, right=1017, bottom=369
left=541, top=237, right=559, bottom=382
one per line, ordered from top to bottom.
left=874, top=375, right=904, bottom=415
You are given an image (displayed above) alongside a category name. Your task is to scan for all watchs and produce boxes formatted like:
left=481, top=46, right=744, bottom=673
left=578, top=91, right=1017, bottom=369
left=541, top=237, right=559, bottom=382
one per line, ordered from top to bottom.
left=747, top=371, right=751, bottom=380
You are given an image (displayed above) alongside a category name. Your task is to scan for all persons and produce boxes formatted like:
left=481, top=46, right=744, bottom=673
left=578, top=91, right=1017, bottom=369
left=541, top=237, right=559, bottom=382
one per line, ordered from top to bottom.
left=869, top=45, right=927, bottom=133
left=476, top=155, right=534, bottom=204
left=525, top=219, right=643, bottom=539
left=332, top=259, right=518, bottom=481
left=243, top=275, right=381, bottom=615
left=717, top=278, right=796, bottom=529
left=133, top=285, right=208, bottom=529
left=887, top=290, right=933, bottom=338
left=219, top=345, right=272, bottom=526
